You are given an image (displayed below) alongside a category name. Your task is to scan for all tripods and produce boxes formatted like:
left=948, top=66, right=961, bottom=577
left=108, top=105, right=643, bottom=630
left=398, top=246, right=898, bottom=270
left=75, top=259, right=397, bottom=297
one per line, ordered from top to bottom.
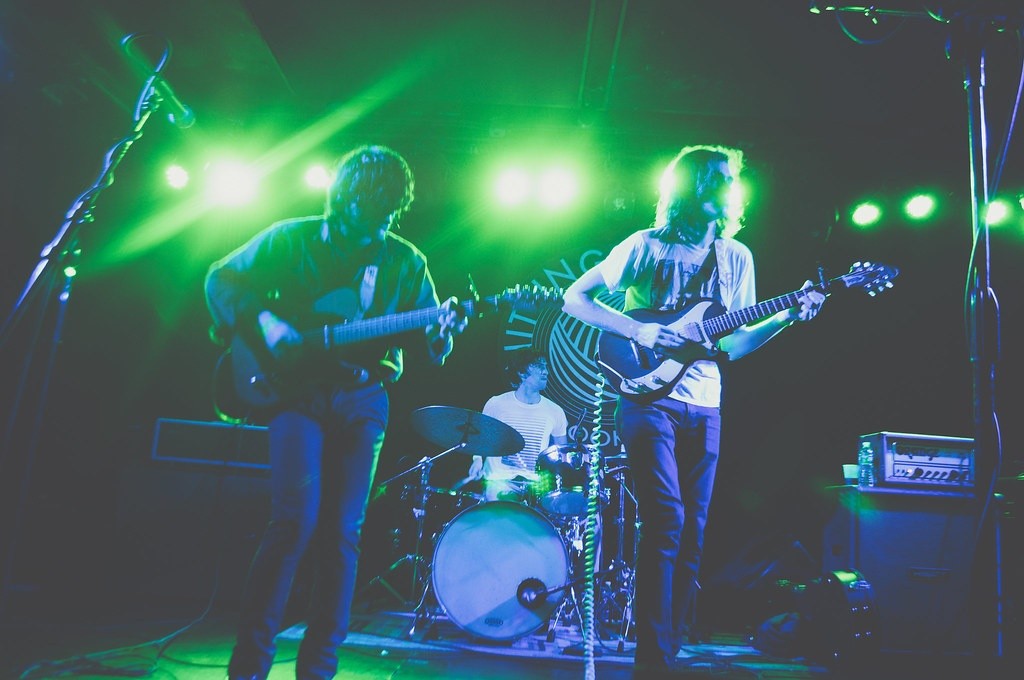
left=352, top=440, right=467, bottom=619
left=565, top=460, right=640, bottom=653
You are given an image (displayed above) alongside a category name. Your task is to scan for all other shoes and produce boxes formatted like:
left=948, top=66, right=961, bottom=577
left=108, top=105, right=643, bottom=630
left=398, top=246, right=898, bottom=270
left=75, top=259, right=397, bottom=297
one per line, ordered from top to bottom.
left=632, top=662, right=712, bottom=678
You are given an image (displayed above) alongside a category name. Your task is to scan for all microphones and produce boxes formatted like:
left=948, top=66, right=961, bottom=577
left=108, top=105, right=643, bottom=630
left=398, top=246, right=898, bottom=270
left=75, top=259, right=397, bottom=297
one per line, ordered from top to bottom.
left=145, top=64, right=195, bottom=128
left=521, top=589, right=536, bottom=606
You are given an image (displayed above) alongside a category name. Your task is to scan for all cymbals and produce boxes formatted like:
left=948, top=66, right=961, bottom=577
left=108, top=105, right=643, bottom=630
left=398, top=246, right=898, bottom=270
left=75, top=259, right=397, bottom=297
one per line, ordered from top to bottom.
left=409, top=405, right=527, bottom=457
left=603, top=452, right=628, bottom=459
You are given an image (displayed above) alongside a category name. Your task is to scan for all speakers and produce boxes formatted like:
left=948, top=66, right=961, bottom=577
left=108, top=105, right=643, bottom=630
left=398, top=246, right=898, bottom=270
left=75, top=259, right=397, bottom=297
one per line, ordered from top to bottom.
left=136, top=467, right=323, bottom=618
left=821, top=485, right=981, bottom=654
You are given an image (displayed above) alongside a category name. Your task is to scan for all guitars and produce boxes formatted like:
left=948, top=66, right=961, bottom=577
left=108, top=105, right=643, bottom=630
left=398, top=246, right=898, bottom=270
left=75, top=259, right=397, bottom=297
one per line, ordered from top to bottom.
left=596, top=260, right=900, bottom=401
left=208, top=269, right=499, bottom=419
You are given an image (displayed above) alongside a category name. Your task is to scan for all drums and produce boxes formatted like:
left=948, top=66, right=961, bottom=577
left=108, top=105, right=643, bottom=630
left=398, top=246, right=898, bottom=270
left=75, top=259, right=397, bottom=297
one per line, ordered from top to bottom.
left=537, top=443, right=609, bottom=516
left=432, top=499, right=573, bottom=645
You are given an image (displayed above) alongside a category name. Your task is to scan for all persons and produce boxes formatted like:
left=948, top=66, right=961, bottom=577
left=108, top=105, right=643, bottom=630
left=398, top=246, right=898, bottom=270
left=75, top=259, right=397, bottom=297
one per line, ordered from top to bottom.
left=203, top=143, right=470, bottom=680
left=560, top=143, right=826, bottom=680
left=468, top=345, right=570, bottom=502
